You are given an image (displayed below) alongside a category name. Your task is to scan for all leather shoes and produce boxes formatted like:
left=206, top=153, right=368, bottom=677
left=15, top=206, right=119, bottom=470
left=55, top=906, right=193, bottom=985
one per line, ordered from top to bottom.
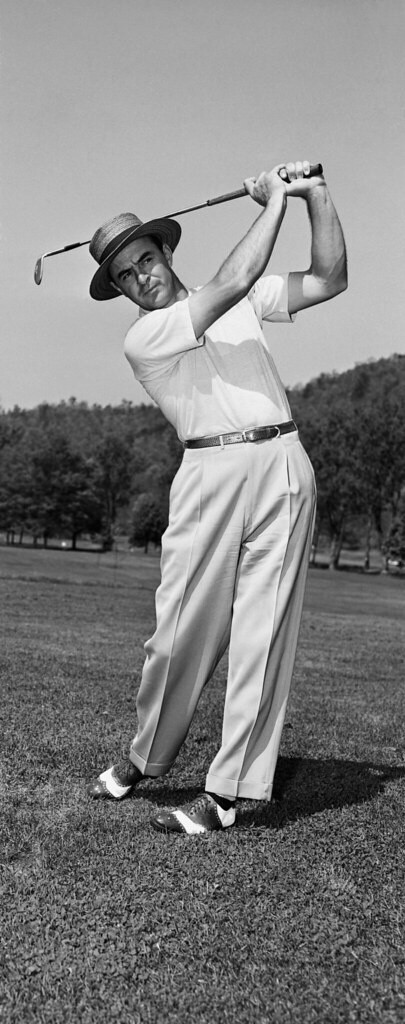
left=86, top=758, right=148, bottom=799
left=149, top=793, right=237, bottom=834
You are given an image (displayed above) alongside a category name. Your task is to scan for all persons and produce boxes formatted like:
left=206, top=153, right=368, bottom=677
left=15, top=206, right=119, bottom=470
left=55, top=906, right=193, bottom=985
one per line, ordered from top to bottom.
left=91, top=161, right=348, bottom=836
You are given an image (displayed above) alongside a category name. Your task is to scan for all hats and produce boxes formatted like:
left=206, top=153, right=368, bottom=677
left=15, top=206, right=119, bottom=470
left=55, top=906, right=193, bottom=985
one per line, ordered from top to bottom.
left=89, top=212, right=181, bottom=302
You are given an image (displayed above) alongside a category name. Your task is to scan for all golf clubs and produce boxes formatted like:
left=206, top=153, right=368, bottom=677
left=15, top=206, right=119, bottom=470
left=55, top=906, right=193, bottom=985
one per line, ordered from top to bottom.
left=34, top=163, right=324, bottom=285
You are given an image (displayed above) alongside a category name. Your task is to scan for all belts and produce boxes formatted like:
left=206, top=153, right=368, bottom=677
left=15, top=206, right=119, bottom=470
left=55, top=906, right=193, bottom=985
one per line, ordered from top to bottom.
left=184, top=421, right=297, bottom=449
